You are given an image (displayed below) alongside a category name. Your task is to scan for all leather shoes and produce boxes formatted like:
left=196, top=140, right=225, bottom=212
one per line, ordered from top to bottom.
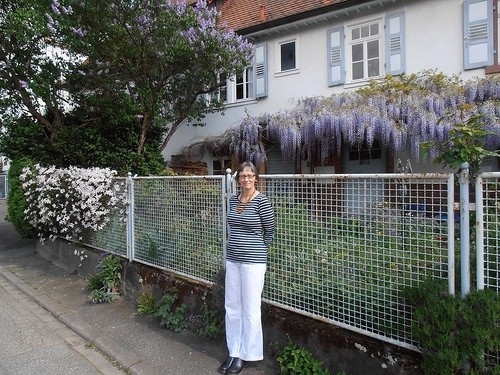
left=219, top=356, right=234, bottom=373
left=227, top=357, right=247, bottom=374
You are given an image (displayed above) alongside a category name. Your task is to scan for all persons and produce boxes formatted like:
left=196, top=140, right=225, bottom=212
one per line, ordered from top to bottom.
left=218, top=161, right=275, bottom=375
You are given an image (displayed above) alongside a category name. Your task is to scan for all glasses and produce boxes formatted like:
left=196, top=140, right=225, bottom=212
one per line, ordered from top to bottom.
left=240, top=173, right=255, bottom=180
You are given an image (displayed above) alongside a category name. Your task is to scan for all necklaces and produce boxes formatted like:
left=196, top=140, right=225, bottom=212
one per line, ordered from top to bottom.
left=238, top=189, right=256, bottom=213
